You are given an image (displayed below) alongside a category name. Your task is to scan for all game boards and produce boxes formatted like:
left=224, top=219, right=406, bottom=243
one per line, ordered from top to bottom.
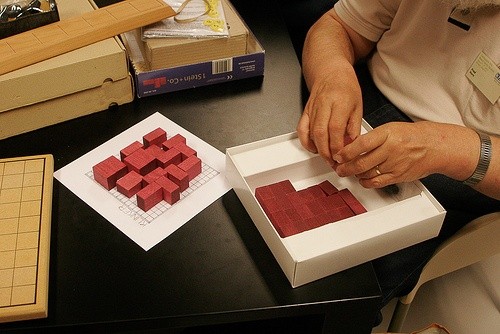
left=1, top=153, right=55, bottom=323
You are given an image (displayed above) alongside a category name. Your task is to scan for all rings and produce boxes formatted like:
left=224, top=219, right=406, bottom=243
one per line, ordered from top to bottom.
left=375, top=165, right=382, bottom=175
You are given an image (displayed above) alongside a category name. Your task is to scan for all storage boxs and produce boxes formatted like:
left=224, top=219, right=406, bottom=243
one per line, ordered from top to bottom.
left=0, top=0, right=129, bottom=114
left=125, top=0, right=248, bottom=71
left=1, top=74, right=134, bottom=141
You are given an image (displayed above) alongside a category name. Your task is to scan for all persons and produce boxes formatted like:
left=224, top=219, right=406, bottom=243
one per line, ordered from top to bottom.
left=297, top=0, right=499, bottom=200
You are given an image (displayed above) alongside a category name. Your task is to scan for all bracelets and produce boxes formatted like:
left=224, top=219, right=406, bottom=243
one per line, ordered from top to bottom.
left=460, top=126, right=492, bottom=187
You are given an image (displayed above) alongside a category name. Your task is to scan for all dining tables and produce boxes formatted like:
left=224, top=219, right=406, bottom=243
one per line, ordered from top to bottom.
left=0, top=22, right=384, bottom=334
